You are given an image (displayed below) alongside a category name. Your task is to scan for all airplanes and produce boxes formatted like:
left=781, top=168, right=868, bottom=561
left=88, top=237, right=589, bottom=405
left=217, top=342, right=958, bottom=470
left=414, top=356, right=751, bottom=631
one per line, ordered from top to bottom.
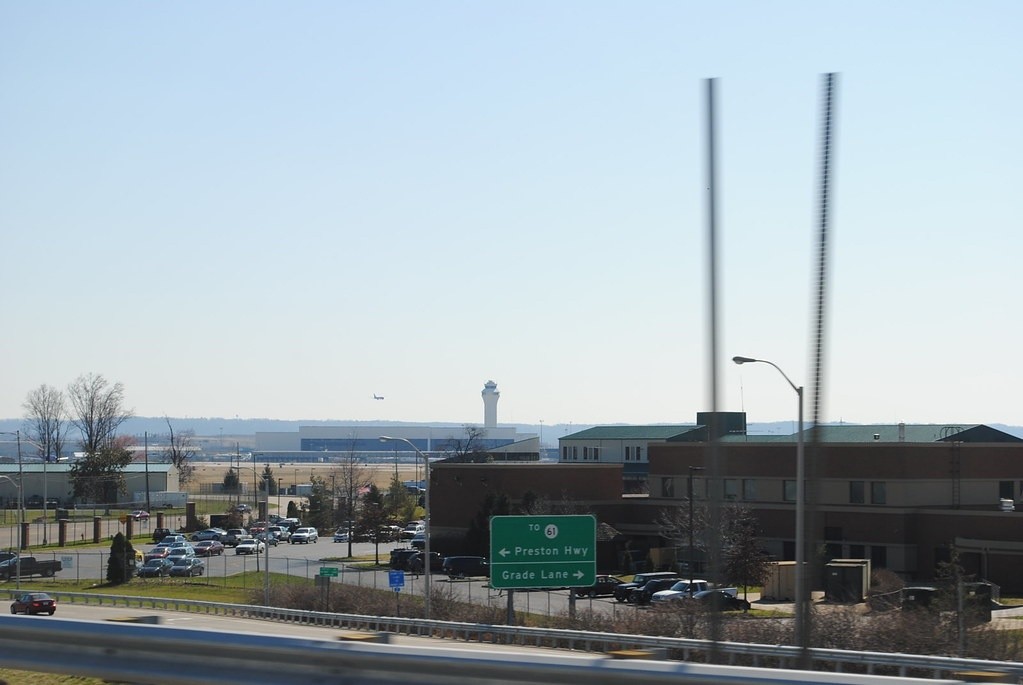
left=373, top=393, right=385, bottom=400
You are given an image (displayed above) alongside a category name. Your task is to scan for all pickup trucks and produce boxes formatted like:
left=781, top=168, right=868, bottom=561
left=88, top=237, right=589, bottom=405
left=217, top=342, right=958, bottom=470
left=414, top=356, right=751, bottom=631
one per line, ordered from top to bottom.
left=0, top=556, right=62, bottom=580
left=650, top=580, right=739, bottom=610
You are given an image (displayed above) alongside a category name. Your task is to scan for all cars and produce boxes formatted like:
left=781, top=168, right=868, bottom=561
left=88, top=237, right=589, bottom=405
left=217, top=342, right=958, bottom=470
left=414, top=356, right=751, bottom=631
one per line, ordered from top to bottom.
left=234, top=503, right=252, bottom=515
left=127, top=510, right=151, bottom=522
left=143, top=514, right=301, bottom=565
left=333, top=520, right=425, bottom=548
left=0, top=551, right=17, bottom=564
left=136, top=558, right=174, bottom=579
left=167, top=558, right=204, bottom=577
left=574, top=574, right=626, bottom=598
left=692, top=590, right=751, bottom=612
left=11, top=593, right=56, bottom=616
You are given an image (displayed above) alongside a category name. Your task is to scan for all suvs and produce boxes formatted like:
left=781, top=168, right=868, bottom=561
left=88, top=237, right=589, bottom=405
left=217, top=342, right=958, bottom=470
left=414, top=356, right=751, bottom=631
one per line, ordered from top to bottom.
left=290, top=527, right=319, bottom=545
left=389, top=548, right=490, bottom=580
left=615, top=572, right=677, bottom=603
left=630, top=577, right=684, bottom=607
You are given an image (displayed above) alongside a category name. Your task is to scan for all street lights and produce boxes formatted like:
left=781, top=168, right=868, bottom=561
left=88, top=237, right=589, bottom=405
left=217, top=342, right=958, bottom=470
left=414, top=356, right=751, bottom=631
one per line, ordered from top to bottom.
left=732, top=356, right=803, bottom=648
left=0, top=430, right=26, bottom=522
left=378, top=436, right=430, bottom=620
left=276, top=450, right=422, bottom=515
left=252, top=452, right=263, bottom=509
left=231, top=466, right=269, bottom=607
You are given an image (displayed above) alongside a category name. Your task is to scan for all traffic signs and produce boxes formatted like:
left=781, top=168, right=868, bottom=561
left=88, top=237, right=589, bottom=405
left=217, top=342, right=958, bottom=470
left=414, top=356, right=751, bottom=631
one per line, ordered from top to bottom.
left=489, top=515, right=597, bottom=589
